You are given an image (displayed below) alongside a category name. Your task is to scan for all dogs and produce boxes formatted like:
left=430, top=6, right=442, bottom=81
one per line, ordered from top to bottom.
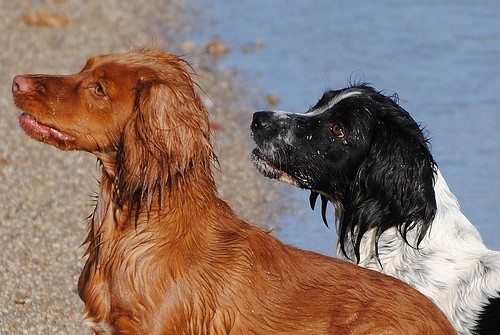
left=11, top=35, right=457, bottom=334
left=249, top=69, right=499, bottom=335
